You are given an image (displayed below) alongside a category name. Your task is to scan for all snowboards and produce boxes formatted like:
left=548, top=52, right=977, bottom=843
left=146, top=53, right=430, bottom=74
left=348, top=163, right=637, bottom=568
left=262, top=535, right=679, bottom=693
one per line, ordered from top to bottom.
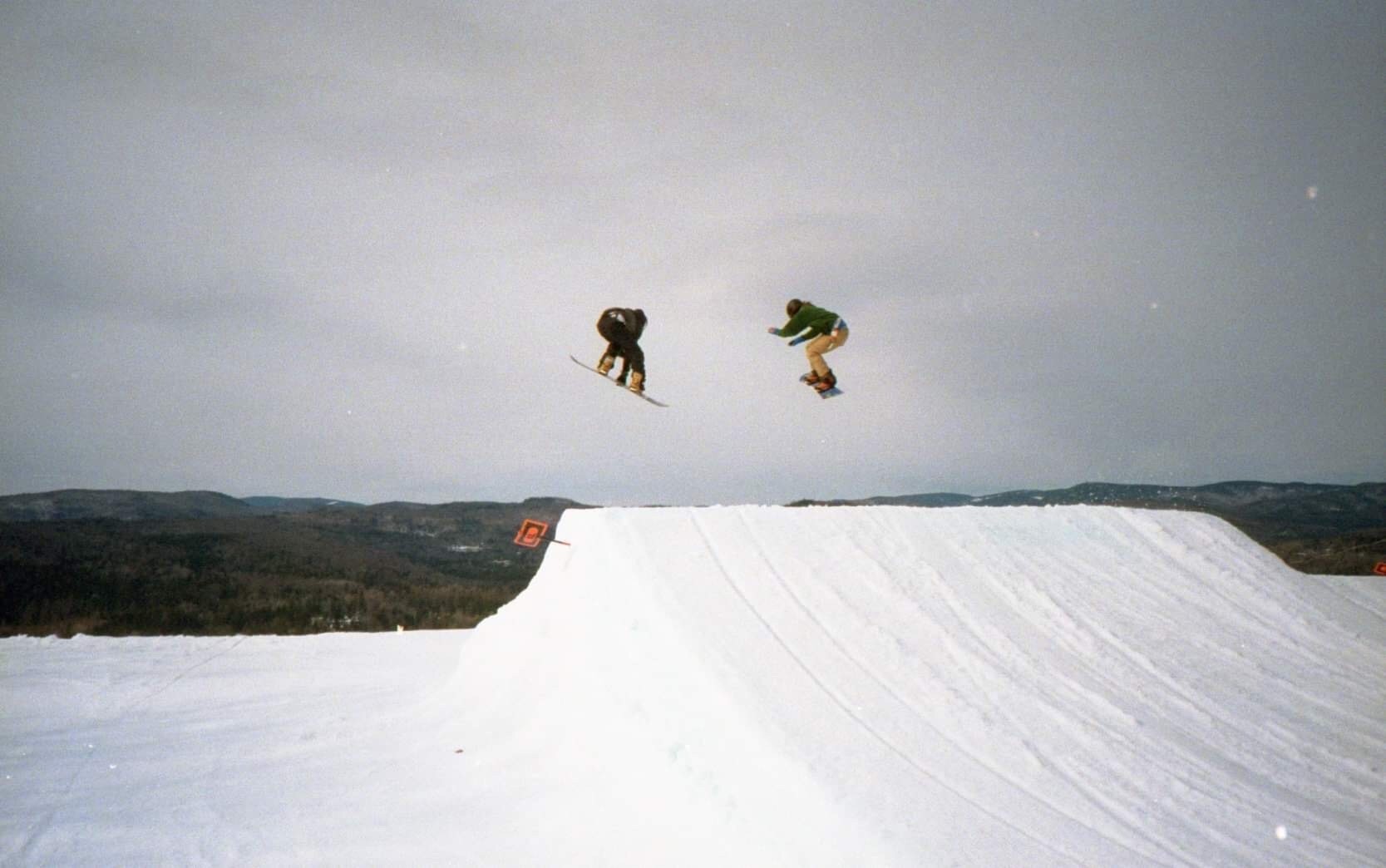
left=569, top=351, right=669, bottom=412
left=800, top=372, right=845, bottom=401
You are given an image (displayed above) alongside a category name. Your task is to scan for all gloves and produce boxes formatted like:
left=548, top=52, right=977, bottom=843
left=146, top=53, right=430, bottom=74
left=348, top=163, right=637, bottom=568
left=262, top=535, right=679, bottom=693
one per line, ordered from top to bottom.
left=789, top=336, right=804, bottom=346
left=616, top=371, right=628, bottom=386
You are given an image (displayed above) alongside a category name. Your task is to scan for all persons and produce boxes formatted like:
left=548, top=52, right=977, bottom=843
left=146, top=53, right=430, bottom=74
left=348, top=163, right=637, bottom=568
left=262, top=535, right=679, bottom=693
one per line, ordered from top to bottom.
left=768, top=299, right=848, bottom=392
left=597, top=308, right=647, bottom=392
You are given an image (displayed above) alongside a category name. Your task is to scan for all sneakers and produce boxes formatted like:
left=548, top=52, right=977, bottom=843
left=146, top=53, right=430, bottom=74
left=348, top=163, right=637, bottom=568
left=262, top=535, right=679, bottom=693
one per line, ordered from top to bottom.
left=814, top=370, right=835, bottom=392
left=804, top=371, right=819, bottom=384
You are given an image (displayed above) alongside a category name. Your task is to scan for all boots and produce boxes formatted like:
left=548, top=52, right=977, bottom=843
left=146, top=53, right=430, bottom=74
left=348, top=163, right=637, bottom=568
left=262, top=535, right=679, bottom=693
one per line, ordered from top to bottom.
left=599, top=355, right=613, bottom=375
left=630, top=372, right=643, bottom=392
